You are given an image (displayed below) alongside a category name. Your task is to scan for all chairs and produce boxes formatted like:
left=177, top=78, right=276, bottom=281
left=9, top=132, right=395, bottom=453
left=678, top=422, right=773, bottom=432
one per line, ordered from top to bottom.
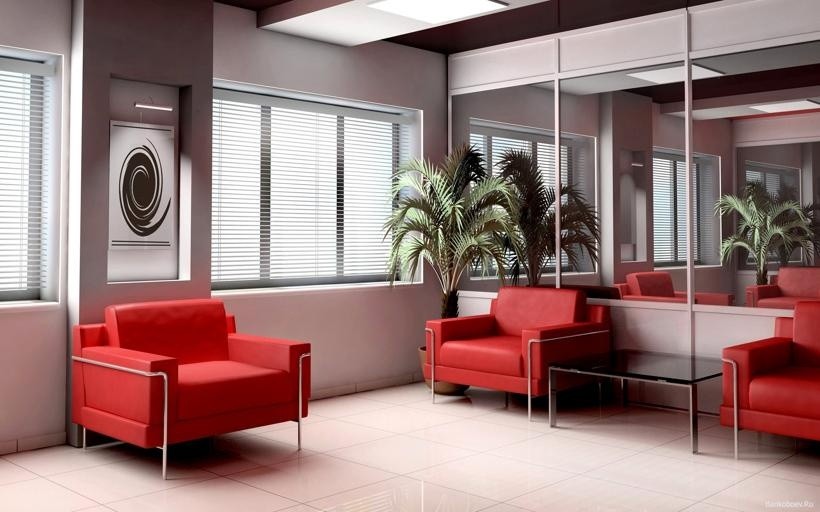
left=745, top=266, right=820, bottom=312
left=617, top=271, right=732, bottom=311
left=425, top=283, right=611, bottom=423
left=72, top=296, right=313, bottom=481
left=722, top=299, right=819, bottom=461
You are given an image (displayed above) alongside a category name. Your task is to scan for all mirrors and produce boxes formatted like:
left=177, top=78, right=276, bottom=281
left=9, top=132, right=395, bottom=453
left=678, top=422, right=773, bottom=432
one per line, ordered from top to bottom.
left=444, top=30, right=820, bottom=319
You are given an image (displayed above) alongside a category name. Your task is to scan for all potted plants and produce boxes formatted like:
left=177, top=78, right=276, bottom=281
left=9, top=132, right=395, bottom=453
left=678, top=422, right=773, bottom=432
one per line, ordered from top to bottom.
left=385, top=145, right=522, bottom=396
